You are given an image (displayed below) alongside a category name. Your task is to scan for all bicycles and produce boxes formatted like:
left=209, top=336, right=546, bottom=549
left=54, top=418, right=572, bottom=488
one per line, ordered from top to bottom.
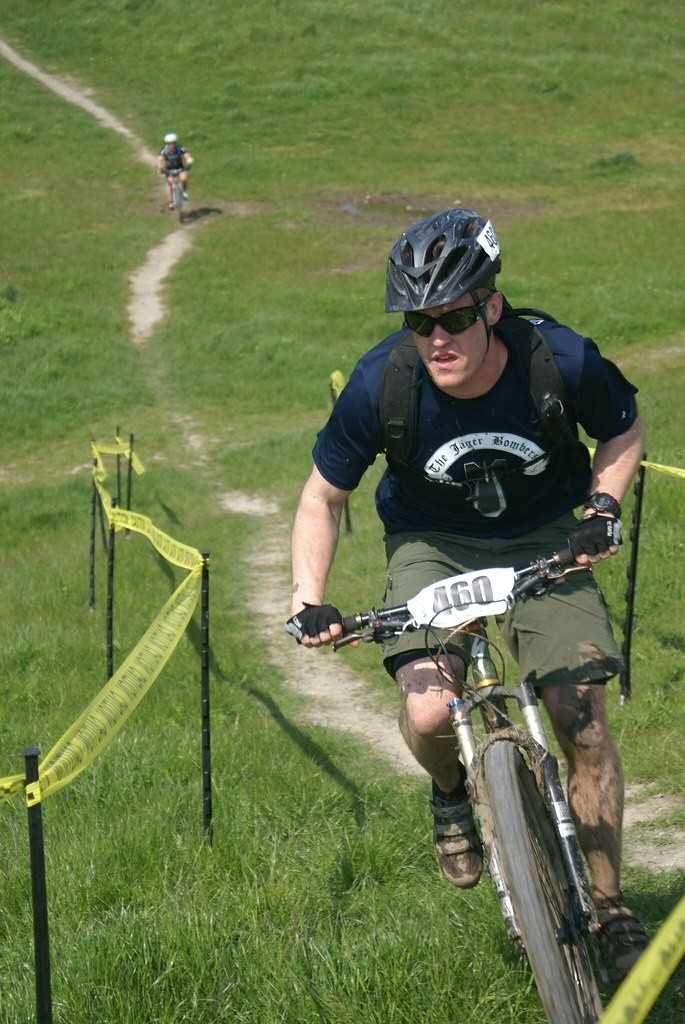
left=160, top=167, right=192, bottom=223
left=296, top=538, right=624, bottom=1023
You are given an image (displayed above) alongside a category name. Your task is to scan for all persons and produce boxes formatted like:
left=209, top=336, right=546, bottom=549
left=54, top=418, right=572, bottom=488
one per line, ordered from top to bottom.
left=158, top=133, right=194, bottom=209
left=286, top=209, right=648, bottom=972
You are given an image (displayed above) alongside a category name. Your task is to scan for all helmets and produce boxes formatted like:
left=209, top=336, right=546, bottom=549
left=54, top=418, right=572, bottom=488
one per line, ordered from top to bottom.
left=163, top=133, right=176, bottom=142
left=385, top=207, right=500, bottom=313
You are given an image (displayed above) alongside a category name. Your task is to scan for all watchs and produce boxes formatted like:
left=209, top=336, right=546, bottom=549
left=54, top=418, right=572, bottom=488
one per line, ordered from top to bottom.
left=583, top=492, right=621, bottom=518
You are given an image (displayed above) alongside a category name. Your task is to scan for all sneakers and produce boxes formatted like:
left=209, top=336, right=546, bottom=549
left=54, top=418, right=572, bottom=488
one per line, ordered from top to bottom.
left=428, top=760, right=484, bottom=888
left=595, top=893, right=649, bottom=983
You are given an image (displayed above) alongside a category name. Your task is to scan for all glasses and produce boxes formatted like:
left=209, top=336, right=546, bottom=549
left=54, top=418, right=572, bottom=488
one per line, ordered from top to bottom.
left=400, top=289, right=493, bottom=336
